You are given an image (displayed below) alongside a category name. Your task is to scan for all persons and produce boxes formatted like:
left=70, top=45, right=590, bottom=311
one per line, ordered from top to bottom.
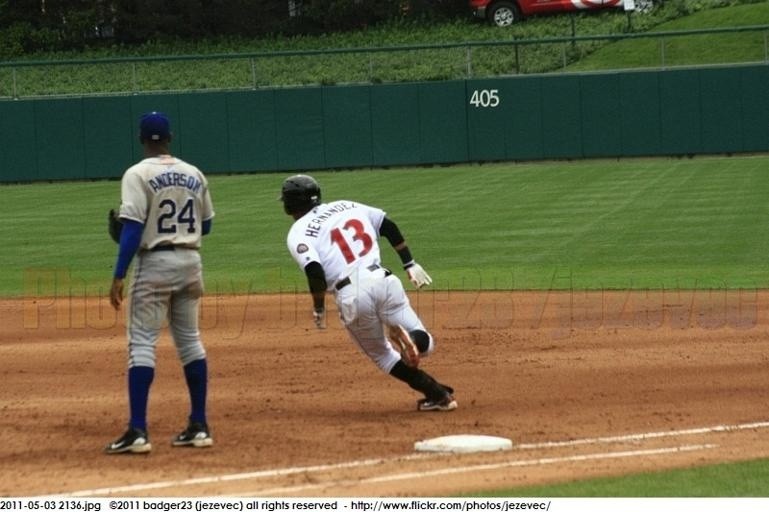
left=106, top=112, right=214, bottom=453
left=278, top=174, right=460, bottom=412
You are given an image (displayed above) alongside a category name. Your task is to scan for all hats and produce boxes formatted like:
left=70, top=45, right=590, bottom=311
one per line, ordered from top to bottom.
left=140, top=111, right=170, bottom=142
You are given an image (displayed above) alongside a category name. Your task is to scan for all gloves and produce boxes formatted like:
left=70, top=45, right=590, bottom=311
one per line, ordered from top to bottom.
left=402, top=259, right=433, bottom=289
left=313, top=311, right=326, bottom=329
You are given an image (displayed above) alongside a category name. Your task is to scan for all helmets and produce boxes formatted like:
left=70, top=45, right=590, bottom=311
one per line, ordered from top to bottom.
left=277, top=174, right=321, bottom=215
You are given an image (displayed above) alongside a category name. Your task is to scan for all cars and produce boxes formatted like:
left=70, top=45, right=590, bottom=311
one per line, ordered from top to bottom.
left=469, top=0, right=653, bottom=28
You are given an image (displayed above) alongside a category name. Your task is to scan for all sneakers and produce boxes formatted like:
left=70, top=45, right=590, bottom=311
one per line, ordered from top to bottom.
left=389, top=324, right=419, bottom=368
left=416, top=390, right=458, bottom=411
left=107, top=427, right=151, bottom=454
left=171, top=429, right=213, bottom=447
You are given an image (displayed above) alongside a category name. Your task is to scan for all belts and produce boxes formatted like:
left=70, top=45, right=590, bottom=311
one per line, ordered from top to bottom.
left=151, top=245, right=174, bottom=250
left=336, top=264, right=379, bottom=290
